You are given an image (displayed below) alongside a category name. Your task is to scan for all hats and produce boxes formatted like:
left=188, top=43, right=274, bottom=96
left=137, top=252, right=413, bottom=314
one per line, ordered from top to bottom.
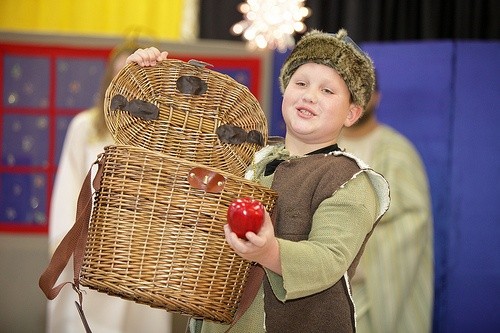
left=280, top=27, right=375, bottom=119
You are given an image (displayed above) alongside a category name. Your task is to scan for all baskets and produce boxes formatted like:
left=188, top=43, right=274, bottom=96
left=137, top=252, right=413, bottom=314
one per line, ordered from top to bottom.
left=39, top=59, right=279, bottom=333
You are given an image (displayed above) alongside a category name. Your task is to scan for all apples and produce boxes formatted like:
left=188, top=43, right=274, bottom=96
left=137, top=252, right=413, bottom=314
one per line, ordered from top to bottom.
left=227, top=197, right=265, bottom=239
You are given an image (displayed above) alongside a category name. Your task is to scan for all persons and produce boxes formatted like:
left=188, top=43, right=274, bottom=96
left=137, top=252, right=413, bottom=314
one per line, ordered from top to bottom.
left=125, top=27, right=391, bottom=333
left=44, top=38, right=174, bottom=332
left=338, top=67, right=435, bottom=332
